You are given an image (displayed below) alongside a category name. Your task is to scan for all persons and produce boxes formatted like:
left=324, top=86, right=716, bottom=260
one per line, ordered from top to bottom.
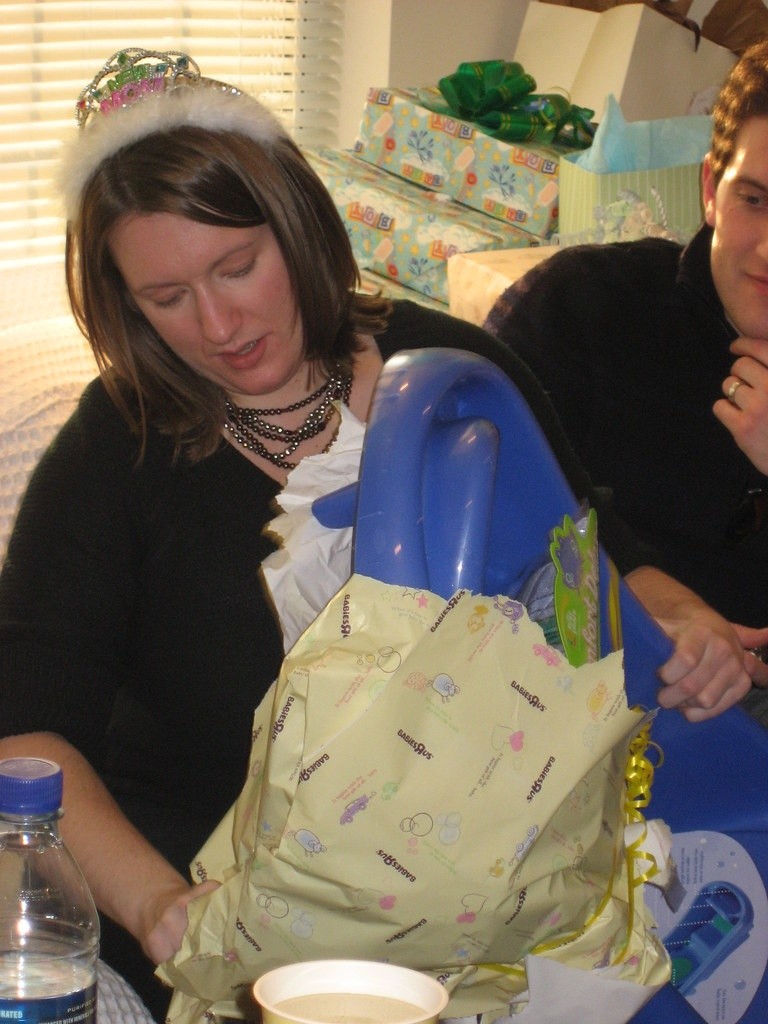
left=488, top=36, right=768, bottom=737
left=1, top=46, right=753, bottom=1024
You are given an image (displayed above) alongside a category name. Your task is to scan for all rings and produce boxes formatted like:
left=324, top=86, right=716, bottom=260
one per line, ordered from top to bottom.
left=727, top=380, right=742, bottom=404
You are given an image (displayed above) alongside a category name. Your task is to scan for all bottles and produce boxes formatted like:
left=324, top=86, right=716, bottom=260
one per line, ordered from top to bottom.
left=0, top=757, right=101, bottom=1024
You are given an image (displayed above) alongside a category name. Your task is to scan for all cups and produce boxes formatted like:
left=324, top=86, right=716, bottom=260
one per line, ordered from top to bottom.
left=253, top=959, right=450, bottom=1024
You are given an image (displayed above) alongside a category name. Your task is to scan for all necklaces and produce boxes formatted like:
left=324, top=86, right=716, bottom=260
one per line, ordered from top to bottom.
left=224, top=360, right=355, bottom=470
